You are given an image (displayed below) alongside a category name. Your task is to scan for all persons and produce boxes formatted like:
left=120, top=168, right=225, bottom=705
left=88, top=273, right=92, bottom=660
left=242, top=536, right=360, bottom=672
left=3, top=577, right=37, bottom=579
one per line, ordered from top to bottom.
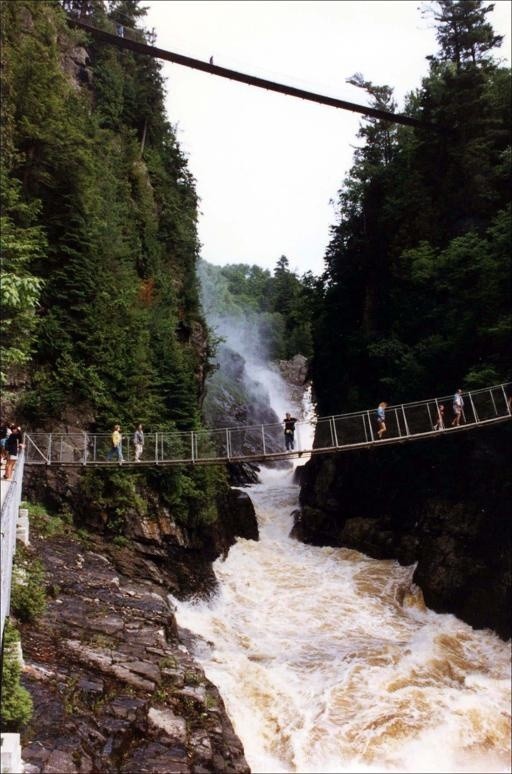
left=115, top=21, right=124, bottom=37
left=209, top=56, right=214, bottom=64
left=451, top=388, right=465, bottom=426
left=375, top=402, right=389, bottom=438
left=433, top=405, right=445, bottom=430
left=282, top=413, right=300, bottom=454
left=0, top=422, right=27, bottom=480
left=133, top=424, right=146, bottom=463
left=106, top=425, right=128, bottom=462
